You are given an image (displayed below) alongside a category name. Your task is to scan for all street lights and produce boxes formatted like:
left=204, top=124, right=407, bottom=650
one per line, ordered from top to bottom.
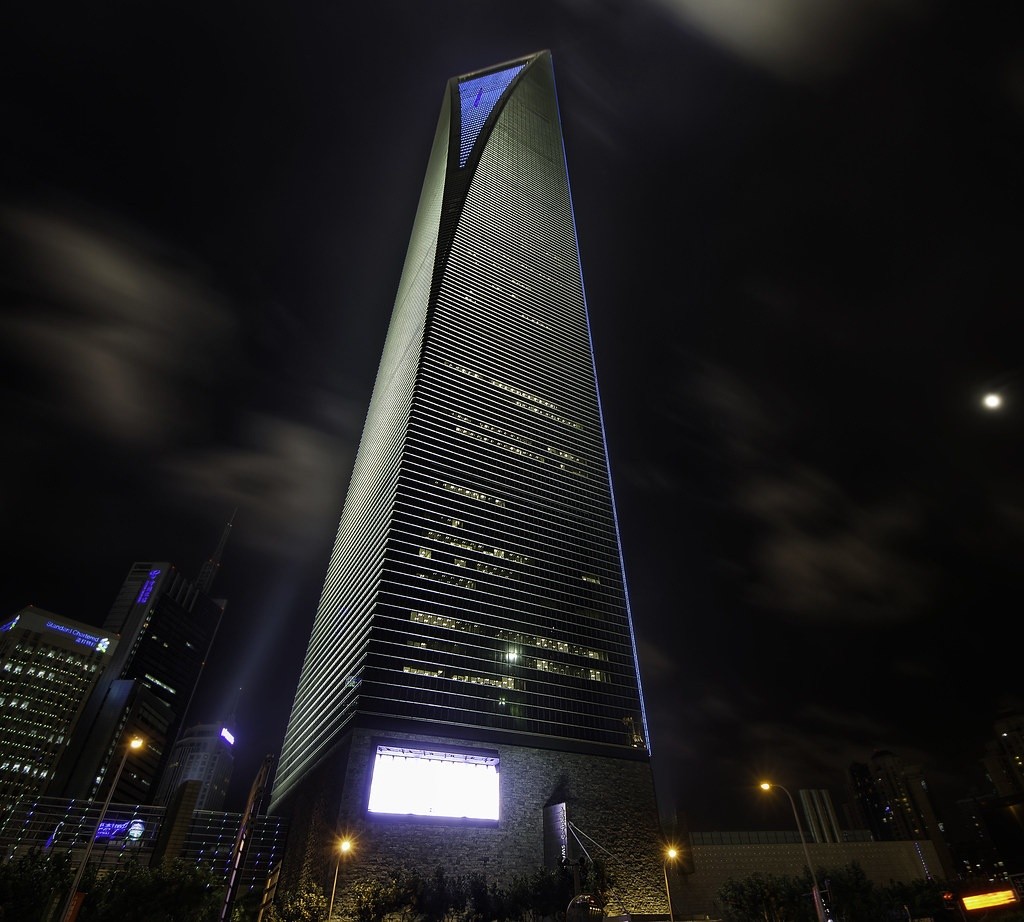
left=52, top=734, right=143, bottom=922
left=760, top=782, right=826, bottom=922
left=328, top=840, right=355, bottom=922
left=663, top=849, right=677, bottom=922
